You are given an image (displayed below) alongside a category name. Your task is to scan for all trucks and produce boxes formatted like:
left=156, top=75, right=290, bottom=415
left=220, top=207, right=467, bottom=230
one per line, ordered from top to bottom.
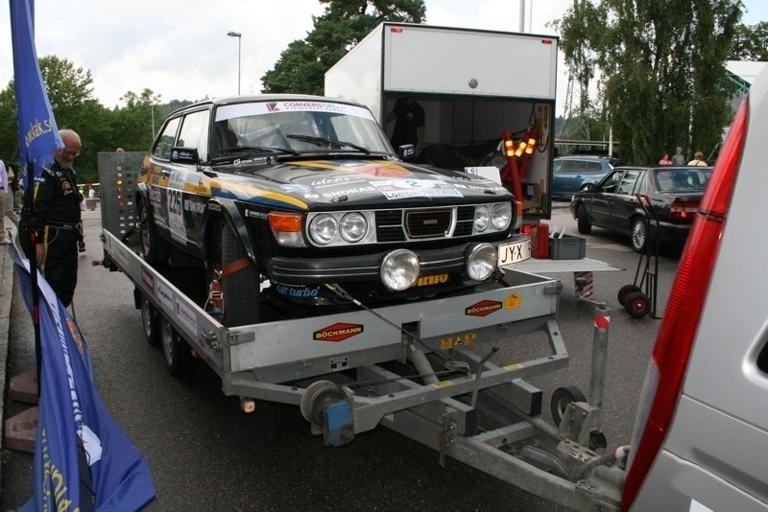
left=100, top=61, right=763, bottom=510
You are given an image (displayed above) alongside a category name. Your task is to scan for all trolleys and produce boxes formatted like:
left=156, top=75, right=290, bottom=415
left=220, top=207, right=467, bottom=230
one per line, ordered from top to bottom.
left=617, top=194, right=665, bottom=319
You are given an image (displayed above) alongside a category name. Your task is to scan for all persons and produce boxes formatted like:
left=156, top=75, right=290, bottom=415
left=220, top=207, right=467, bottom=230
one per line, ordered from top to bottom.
left=383, top=95, right=426, bottom=158
left=0, top=128, right=82, bottom=308
left=672, top=146, right=685, bottom=166
left=688, top=151, right=709, bottom=167
left=657, top=153, right=672, bottom=165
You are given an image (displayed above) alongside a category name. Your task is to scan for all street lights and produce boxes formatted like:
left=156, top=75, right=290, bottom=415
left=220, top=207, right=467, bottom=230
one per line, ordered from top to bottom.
left=227, top=31, right=242, bottom=94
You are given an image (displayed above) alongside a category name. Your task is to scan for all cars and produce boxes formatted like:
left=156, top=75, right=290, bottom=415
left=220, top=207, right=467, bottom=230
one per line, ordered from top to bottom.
left=551, top=155, right=624, bottom=201
left=572, top=164, right=715, bottom=258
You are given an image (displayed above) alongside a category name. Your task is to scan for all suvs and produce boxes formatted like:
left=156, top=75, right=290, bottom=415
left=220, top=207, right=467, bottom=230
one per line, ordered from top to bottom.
left=135, top=93, right=532, bottom=326
